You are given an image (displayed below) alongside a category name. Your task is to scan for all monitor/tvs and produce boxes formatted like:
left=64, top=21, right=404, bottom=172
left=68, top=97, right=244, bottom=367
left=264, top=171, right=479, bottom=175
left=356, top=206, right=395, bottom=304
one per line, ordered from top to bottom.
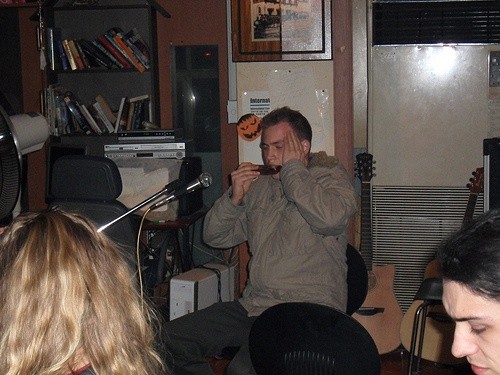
left=45, top=144, right=89, bottom=198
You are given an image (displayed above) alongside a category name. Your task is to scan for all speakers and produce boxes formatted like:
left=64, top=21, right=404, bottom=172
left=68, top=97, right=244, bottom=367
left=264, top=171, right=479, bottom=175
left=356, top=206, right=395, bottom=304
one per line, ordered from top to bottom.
left=174, top=43, right=222, bottom=265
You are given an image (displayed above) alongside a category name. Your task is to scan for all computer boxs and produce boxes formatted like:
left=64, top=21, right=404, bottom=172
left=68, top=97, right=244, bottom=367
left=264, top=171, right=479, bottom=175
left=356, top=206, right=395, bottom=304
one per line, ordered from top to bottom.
left=168, top=261, right=235, bottom=323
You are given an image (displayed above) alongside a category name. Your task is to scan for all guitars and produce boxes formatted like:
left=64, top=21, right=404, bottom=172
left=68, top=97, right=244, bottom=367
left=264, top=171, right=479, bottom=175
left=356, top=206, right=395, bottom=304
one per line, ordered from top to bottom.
left=350, top=152, right=405, bottom=356
left=401, top=165, right=485, bottom=366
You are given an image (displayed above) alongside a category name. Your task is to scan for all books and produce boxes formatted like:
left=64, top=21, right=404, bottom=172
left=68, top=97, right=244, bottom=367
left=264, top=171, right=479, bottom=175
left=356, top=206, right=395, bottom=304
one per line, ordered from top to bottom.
left=47, top=27, right=150, bottom=135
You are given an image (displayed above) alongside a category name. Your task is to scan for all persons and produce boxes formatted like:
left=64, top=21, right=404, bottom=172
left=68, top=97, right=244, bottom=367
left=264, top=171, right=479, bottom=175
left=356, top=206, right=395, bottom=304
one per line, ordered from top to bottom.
left=158, top=108, right=357, bottom=375
left=0, top=206, right=176, bottom=375
left=439, top=209, right=500, bottom=375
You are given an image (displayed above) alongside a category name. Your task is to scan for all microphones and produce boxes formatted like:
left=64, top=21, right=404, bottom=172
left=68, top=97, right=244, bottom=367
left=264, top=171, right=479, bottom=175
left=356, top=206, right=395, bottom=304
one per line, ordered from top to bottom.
left=150, top=173, right=213, bottom=209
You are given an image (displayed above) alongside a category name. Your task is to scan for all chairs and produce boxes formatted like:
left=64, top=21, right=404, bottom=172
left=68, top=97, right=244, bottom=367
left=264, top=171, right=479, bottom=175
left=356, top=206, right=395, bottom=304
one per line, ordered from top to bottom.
left=48, top=155, right=139, bottom=279
left=345, top=243, right=369, bottom=316
left=408, top=278, right=455, bottom=375
left=248, top=302, right=382, bottom=375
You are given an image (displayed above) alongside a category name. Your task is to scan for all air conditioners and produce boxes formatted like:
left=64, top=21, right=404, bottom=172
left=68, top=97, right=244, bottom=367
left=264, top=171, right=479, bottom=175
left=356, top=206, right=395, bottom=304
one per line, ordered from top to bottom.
left=365, top=0, right=500, bottom=313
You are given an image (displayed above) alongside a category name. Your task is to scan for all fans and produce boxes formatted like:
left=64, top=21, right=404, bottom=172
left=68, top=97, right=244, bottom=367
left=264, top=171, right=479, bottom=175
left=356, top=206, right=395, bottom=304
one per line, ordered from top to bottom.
left=0, top=104, right=50, bottom=221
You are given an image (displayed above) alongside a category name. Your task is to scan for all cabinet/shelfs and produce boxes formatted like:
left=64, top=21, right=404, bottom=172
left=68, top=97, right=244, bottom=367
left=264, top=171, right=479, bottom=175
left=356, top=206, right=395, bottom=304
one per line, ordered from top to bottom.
left=42, top=0, right=159, bottom=154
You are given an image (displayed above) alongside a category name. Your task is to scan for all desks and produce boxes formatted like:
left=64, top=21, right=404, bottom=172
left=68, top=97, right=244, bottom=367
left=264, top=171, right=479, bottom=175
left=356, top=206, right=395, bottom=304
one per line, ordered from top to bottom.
left=138, top=207, right=209, bottom=285
